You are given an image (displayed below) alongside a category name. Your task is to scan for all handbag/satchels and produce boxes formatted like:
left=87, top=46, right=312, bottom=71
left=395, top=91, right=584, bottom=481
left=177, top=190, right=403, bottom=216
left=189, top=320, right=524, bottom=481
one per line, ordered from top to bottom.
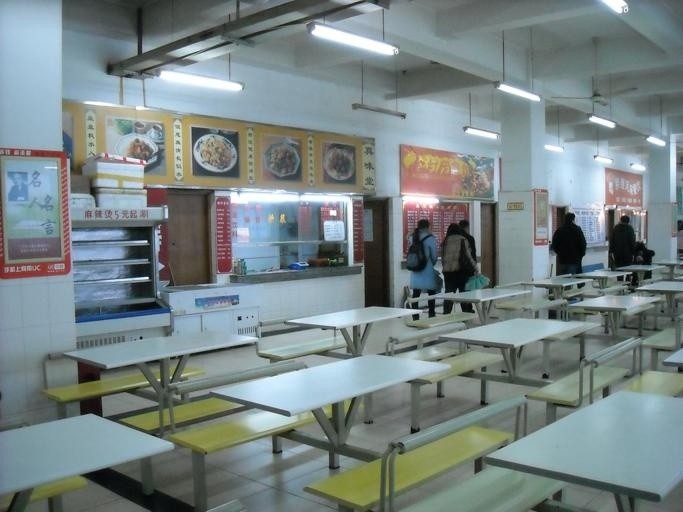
left=451, top=255, right=474, bottom=281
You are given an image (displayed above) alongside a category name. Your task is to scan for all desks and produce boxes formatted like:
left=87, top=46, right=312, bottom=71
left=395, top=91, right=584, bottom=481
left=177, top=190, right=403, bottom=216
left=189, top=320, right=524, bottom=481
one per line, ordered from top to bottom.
left=282, top=258, right=683, bottom=422
left=62, top=332, right=262, bottom=397
left=208, top=352, right=451, bottom=451
left=483, top=390, right=683, bottom=512
left=0, top=412, right=176, bottom=496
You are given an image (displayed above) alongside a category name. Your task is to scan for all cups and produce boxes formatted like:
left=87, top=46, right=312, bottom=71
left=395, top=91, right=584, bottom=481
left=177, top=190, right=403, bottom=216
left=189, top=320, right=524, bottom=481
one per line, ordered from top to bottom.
left=134, top=121, right=146, bottom=135
left=150, top=124, right=163, bottom=140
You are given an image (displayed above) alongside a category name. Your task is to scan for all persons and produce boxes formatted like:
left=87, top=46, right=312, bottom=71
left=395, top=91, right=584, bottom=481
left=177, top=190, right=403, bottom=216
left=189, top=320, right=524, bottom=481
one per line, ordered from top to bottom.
left=552, top=212, right=587, bottom=301
left=607, top=215, right=636, bottom=295
left=409, top=218, right=443, bottom=321
left=458, top=220, right=477, bottom=264
left=677, top=220, right=683, bottom=269
left=440, top=222, right=479, bottom=315
left=631, top=241, right=656, bottom=292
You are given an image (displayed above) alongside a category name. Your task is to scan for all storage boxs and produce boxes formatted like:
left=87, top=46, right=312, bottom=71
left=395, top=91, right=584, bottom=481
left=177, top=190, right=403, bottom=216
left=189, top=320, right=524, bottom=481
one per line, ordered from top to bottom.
left=80, top=160, right=147, bottom=189
left=68, top=173, right=92, bottom=193
left=91, top=188, right=148, bottom=208
left=69, top=192, right=97, bottom=209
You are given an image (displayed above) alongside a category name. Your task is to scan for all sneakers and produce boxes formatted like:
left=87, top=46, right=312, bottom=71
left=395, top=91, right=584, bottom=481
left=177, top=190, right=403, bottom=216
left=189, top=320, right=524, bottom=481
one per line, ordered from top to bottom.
left=577, top=282, right=585, bottom=289
left=628, top=285, right=636, bottom=291
left=461, top=309, right=475, bottom=314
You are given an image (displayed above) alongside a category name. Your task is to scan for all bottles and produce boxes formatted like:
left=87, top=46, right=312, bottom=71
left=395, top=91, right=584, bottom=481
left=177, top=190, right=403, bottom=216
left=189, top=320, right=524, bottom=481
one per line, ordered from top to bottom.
left=234, top=258, right=247, bottom=275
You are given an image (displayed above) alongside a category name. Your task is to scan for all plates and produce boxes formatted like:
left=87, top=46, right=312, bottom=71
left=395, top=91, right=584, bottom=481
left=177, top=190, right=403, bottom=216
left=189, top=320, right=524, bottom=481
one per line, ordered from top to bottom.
left=145, top=130, right=164, bottom=144
left=114, top=132, right=158, bottom=168
left=193, top=133, right=237, bottom=173
left=262, top=143, right=300, bottom=178
left=323, top=146, right=354, bottom=182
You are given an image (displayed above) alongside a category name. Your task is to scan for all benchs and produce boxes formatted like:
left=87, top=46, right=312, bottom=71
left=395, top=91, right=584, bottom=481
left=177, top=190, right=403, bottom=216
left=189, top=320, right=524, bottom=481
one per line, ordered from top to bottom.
left=258, top=336, right=351, bottom=368
left=39, top=366, right=210, bottom=412
left=119, top=397, right=250, bottom=497
left=166, top=397, right=368, bottom=511
left=300, top=422, right=519, bottom=512
left=395, top=465, right=569, bottom=511
left=1, top=474, right=89, bottom=512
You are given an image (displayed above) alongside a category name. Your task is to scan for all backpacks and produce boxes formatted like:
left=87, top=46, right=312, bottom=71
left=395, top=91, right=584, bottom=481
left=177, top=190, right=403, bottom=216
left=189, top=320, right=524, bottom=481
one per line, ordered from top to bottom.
left=404, top=232, right=433, bottom=273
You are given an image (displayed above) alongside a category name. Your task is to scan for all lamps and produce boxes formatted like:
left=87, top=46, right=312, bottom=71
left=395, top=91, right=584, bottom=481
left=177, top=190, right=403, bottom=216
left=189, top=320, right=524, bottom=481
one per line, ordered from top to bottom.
left=597, top=1, right=633, bottom=17
left=306, top=8, right=405, bottom=60
left=153, top=12, right=249, bottom=97
left=490, top=28, right=547, bottom=109
left=350, top=60, right=412, bottom=123
left=585, top=78, right=618, bottom=132
left=459, top=92, right=504, bottom=144
left=543, top=106, right=666, bottom=174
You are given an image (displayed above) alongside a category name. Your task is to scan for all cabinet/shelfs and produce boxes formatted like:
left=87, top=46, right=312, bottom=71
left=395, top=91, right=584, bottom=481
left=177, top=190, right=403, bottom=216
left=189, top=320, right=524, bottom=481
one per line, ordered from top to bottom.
left=67, top=205, right=170, bottom=351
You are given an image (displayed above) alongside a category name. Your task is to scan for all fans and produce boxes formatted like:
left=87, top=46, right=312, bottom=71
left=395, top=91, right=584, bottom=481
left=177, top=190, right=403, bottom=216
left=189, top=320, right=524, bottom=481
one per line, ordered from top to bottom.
left=550, top=74, right=640, bottom=111
